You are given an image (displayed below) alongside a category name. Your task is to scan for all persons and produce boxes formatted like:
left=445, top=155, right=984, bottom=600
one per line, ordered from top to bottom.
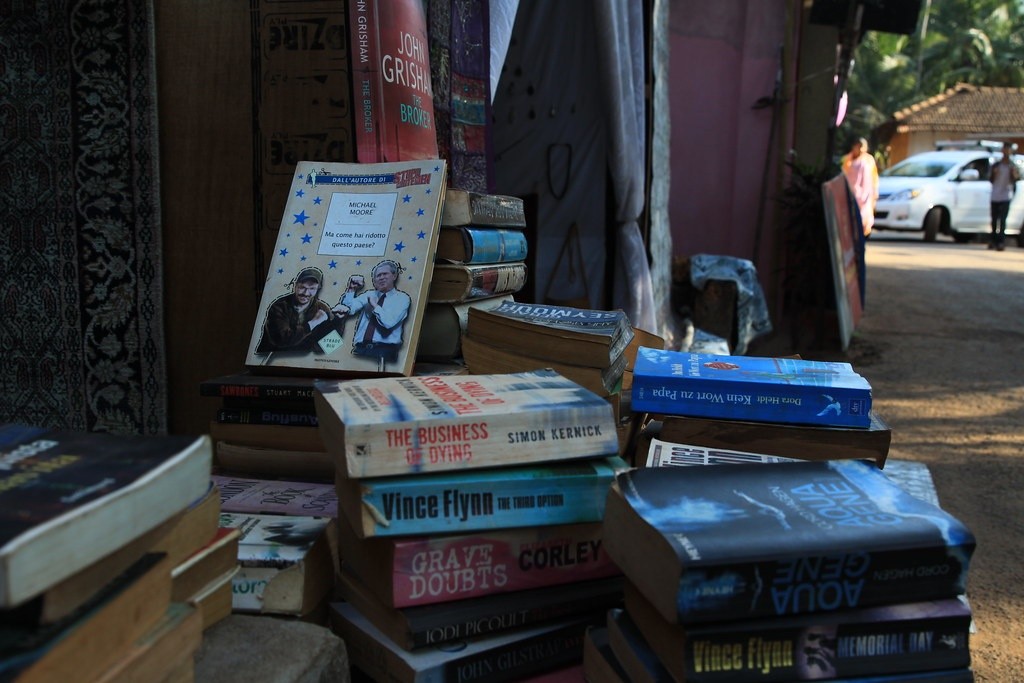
left=838, top=137, right=877, bottom=242
left=988, top=142, right=1022, bottom=250
left=338, top=260, right=412, bottom=364
left=257, top=262, right=346, bottom=364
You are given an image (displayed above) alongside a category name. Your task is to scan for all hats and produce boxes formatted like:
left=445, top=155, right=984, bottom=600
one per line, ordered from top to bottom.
left=296, top=269, right=322, bottom=285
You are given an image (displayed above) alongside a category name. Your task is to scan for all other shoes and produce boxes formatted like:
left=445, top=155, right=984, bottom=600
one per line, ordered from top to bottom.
left=988, top=238, right=996, bottom=249
left=997, top=239, right=1004, bottom=250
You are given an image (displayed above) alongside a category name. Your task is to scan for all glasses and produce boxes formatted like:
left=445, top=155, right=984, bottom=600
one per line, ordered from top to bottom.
left=296, top=286, right=316, bottom=291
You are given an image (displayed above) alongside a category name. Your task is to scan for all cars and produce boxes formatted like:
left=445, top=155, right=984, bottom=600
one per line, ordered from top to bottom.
left=865, top=139, right=1024, bottom=245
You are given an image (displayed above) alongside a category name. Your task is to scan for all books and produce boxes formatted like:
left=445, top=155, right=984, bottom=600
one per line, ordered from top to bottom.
left=0, top=0, right=976, bottom=683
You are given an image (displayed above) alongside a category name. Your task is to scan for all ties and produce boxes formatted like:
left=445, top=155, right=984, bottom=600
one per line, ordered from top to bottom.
left=363, top=293, right=386, bottom=343
